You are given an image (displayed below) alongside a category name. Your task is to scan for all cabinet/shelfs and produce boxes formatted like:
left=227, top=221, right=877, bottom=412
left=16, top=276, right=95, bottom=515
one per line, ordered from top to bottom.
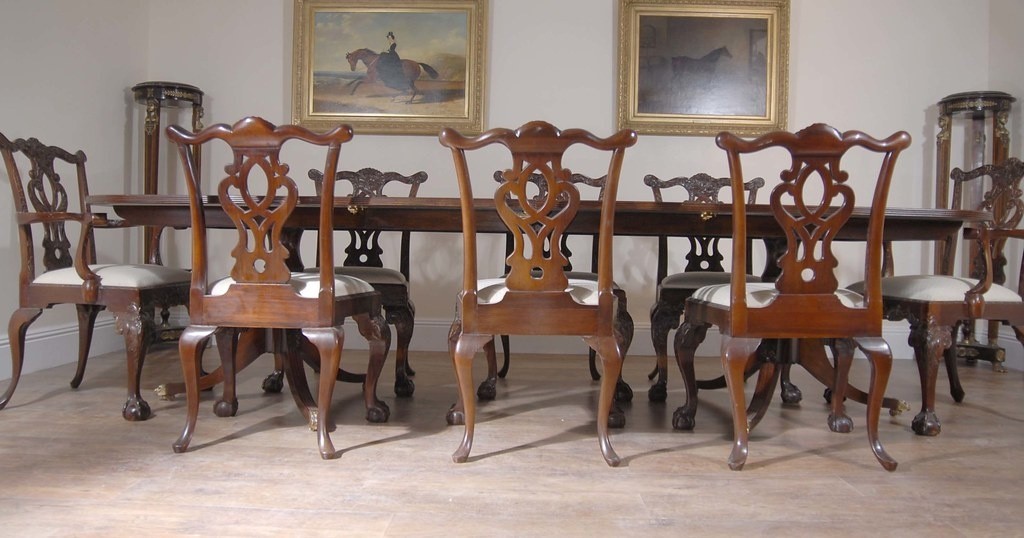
left=131, top=79, right=205, bottom=352
left=934, top=90, right=1016, bottom=372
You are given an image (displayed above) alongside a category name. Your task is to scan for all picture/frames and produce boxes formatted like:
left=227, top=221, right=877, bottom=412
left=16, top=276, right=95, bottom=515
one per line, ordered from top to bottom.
left=292, top=0, right=487, bottom=137
left=615, top=0, right=791, bottom=139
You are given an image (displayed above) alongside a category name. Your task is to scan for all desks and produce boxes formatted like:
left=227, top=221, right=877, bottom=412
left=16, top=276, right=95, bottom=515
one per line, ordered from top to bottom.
left=85, top=189, right=994, bottom=417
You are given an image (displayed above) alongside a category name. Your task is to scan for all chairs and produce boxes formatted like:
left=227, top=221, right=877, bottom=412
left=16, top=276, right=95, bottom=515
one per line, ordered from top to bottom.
left=162, top=117, right=393, bottom=460
left=439, top=119, right=639, bottom=466
left=675, top=125, right=914, bottom=471
left=0, top=132, right=195, bottom=422
left=823, top=157, right=1024, bottom=435
left=474, top=168, right=637, bottom=401
left=259, top=170, right=428, bottom=395
left=644, top=174, right=802, bottom=404
